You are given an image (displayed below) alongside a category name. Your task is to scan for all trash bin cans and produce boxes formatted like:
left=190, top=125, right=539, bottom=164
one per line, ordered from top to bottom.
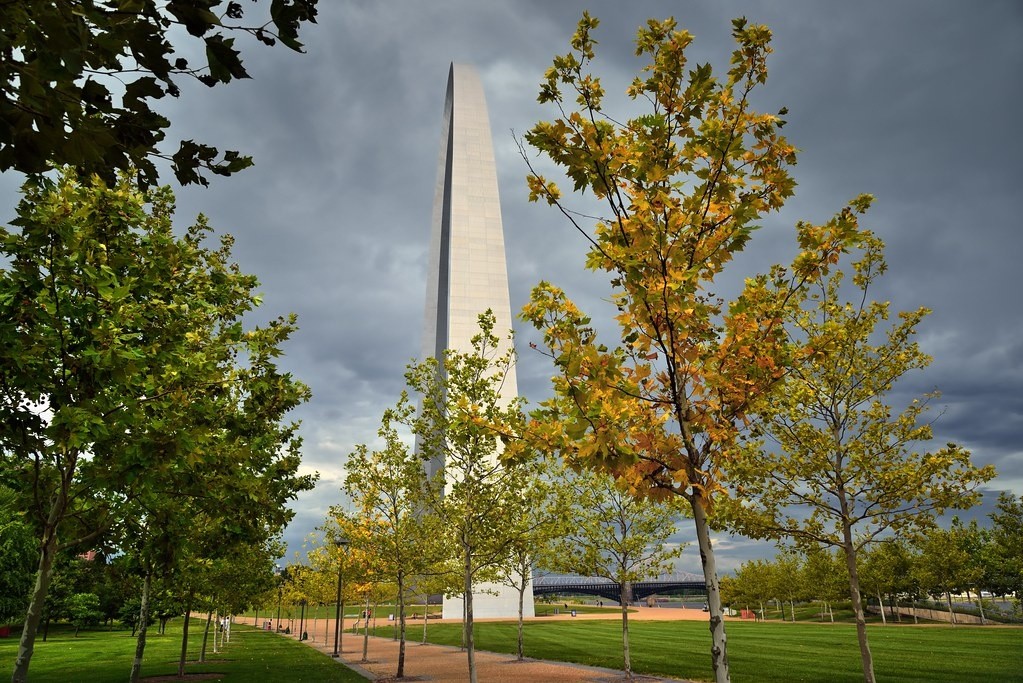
left=303, top=632, right=308, bottom=640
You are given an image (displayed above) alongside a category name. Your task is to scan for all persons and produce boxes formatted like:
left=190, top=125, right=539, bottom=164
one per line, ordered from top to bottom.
left=596, top=599, right=599, bottom=607
left=600, top=601, right=604, bottom=608
left=564, top=603, right=568, bottom=611
left=368, top=609, right=371, bottom=621
left=363, top=610, right=366, bottom=618
left=219, top=617, right=225, bottom=632
left=225, top=617, right=229, bottom=630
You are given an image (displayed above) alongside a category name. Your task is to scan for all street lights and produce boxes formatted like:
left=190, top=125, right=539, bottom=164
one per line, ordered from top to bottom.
left=331, top=537, right=350, bottom=658
left=275, top=585, right=285, bottom=633
